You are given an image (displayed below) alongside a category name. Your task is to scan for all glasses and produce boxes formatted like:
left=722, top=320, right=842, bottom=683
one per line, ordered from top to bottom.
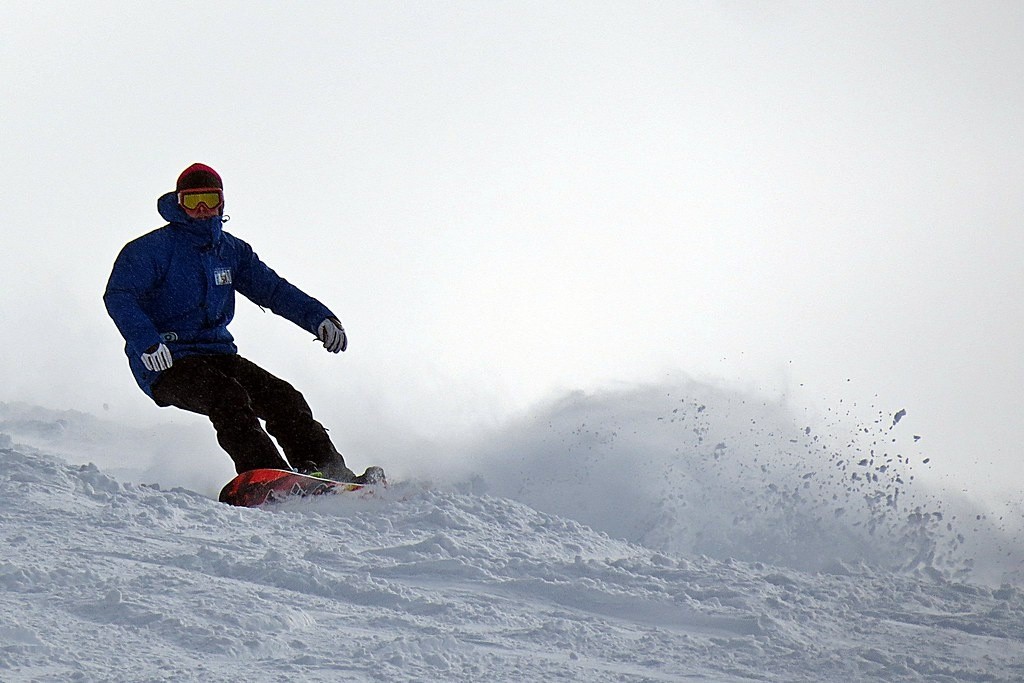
left=179, top=190, right=223, bottom=211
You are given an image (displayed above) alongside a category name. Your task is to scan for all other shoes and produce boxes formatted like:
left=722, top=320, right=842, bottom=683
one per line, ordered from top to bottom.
left=296, top=462, right=322, bottom=478
left=350, top=467, right=386, bottom=484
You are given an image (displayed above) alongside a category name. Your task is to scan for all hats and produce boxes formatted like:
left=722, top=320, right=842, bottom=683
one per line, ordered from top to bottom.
left=176, top=163, right=223, bottom=193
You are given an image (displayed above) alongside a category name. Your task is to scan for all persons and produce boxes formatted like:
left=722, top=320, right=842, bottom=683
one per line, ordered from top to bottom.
left=102, top=164, right=356, bottom=481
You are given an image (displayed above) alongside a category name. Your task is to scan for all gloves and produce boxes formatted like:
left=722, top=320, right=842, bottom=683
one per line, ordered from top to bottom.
left=318, top=318, right=348, bottom=353
left=140, top=342, right=173, bottom=372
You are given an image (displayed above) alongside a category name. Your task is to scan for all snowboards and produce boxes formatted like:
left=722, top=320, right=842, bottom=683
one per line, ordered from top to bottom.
left=218, top=468, right=389, bottom=508
